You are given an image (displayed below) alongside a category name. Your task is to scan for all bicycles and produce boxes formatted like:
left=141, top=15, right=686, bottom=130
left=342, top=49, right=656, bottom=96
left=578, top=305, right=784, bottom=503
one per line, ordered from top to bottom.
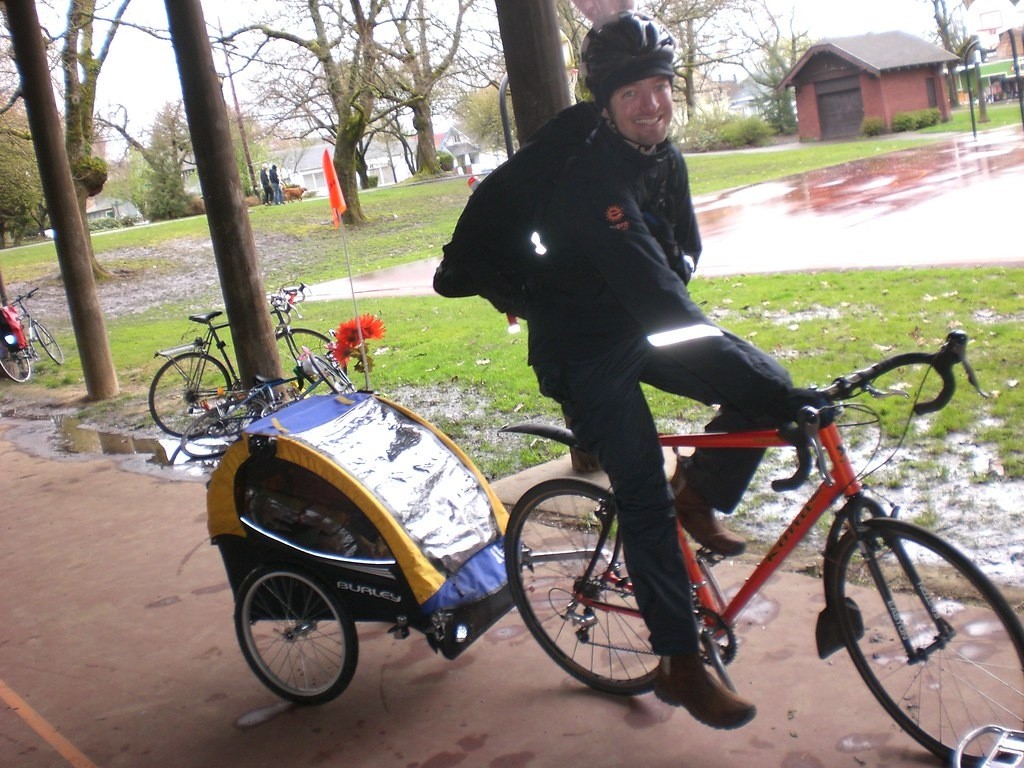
left=148, top=280, right=349, bottom=440
left=0, top=287, right=65, bottom=384
left=203, top=328, right=1024, bottom=765
left=177, top=351, right=357, bottom=462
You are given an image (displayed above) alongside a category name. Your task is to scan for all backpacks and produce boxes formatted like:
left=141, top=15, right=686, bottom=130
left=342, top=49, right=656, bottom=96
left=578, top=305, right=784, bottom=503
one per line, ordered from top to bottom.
left=433, top=101, right=618, bottom=319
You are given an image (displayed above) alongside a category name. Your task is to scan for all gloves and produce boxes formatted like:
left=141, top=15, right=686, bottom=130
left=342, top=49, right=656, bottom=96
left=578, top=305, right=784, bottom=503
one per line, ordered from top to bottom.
left=645, top=176, right=680, bottom=258
left=777, top=388, right=835, bottom=428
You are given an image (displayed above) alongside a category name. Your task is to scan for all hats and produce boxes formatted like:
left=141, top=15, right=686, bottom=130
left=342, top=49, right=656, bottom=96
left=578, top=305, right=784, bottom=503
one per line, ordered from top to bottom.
left=262, top=163, right=268, bottom=169
left=272, top=165, right=276, bottom=169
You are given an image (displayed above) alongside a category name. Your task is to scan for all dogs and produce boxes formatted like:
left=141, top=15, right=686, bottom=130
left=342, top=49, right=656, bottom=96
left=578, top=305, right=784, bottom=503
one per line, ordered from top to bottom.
left=284, top=188, right=308, bottom=203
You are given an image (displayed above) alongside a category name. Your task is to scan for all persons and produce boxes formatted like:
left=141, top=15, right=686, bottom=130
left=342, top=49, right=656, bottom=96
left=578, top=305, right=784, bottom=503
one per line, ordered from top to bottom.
left=522, top=9, right=835, bottom=729
left=260, top=163, right=274, bottom=206
left=270, top=164, right=286, bottom=204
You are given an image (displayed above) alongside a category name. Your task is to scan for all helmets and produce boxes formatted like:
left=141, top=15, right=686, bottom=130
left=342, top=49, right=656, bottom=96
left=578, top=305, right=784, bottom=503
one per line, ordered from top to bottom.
left=581, top=10, right=677, bottom=92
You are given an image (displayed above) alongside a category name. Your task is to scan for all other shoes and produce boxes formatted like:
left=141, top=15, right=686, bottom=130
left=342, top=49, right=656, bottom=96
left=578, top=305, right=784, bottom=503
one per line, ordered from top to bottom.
left=667, top=454, right=747, bottom=555
left=654, top=652, right=757, bottom=730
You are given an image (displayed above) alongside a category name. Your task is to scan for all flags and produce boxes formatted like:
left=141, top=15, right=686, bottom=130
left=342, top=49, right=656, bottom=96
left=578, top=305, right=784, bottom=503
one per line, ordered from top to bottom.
left=322, top=149, right=346, bottom=229
